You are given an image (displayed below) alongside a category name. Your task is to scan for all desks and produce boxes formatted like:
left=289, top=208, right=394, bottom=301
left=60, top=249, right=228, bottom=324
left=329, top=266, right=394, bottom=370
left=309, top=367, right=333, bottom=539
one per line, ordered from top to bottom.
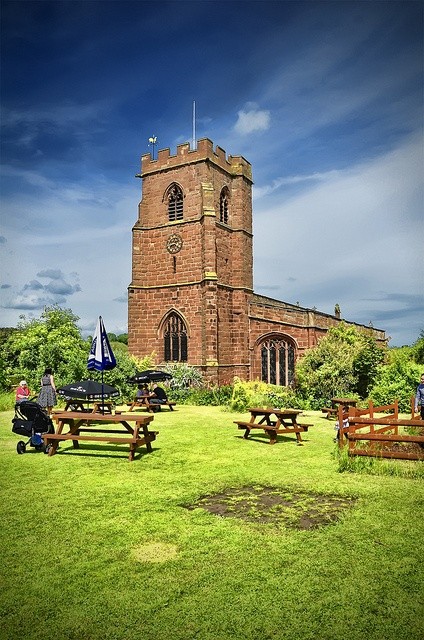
left=128, top=395, right=155, bottom=413
left=326, top=398, right=357, bottom=420
left=62, top=400, right=113, bottom=414
left=243, top=407, right=302, bottom=444
left=48, top=413, right=154, bottom=461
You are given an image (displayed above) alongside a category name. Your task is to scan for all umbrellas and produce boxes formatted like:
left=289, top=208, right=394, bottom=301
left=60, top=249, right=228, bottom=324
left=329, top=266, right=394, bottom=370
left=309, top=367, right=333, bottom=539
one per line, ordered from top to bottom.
left=87, top=316, right=117, bottom=416
left=126, top=370, right=173, bottom=384
left=56, top=381, right=120, bottom=426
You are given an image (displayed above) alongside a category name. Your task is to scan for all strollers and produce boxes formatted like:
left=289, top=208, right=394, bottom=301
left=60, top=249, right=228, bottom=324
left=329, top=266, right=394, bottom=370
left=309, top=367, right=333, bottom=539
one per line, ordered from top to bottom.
left=12, top=396, right=55, bottom=454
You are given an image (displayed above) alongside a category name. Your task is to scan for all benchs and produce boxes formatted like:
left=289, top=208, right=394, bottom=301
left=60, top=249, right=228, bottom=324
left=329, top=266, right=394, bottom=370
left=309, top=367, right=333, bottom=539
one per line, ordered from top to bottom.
left=126, top=402, right=146, bottom=406
left=141, top=402, right=176, bottom=411
left=83, top=408, right=110, bottom=412
left=321, top=408, right=336, bottom=413
left=232, top=420, right=279, bottom=431
left=267, top=421, right=314, bottom=428
left=78, top=429, right=160, bottom=434
left=41, top=434, right=143, bottom=446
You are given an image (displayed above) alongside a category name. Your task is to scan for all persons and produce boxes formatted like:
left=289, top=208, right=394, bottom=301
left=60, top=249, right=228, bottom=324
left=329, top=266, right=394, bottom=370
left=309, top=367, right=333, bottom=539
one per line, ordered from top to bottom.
left=36, top=367, right=57, bottom=415
left=414, top=373, right=424, bottom=420
left=16, top=380, right=31, bottom=404
left=143, top=383, right=149, bottom=395
left=148, top=383, right=167, bottom=413
left=136, top=385, right=155, bottom=413
left=334, top=405, right=349, bottom=440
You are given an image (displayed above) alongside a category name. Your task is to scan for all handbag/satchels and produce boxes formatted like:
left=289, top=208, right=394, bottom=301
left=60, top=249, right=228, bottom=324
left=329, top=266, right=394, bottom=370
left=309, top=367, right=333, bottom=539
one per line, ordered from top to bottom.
left=12, top=416, right=34, bottom=436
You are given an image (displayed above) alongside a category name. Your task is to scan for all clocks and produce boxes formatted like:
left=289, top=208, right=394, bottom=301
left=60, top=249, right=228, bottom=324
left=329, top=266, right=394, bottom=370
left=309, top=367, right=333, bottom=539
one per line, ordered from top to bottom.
left=165, top=234, right=183, bottom=254
